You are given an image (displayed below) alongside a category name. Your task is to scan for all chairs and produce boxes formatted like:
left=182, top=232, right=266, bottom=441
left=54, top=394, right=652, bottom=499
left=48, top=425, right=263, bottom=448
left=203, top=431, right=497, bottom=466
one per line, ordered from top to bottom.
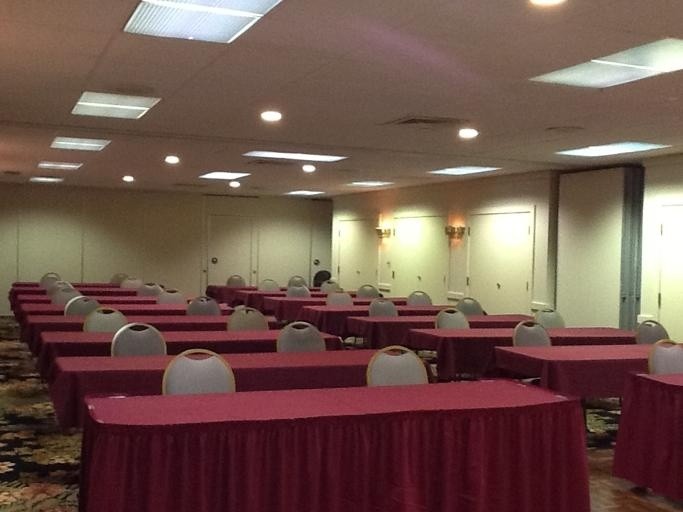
left=39, top=272, right=61, bottom=288
left=111, top=272, right=128, bottom=283
left=227, top=273, right=246, bottom=286
left=287, top=275, right=306, bottom=287
left=120, top=276, right=143, bottom=288
left=257, top=278, right=279, bottom=292
left=320, top=279, right=342, bottom=293
left=46, top=281, right=75, bottom=296
left=136, top=281, right=164, bottom=296
left=287, top=284, right=310, bottom=298
left=356, top=284, right=379, bottom=299
left=52, top=287, right=82, bottom=303
left=326, top=287, right=353, bottom=305
left=156, top=288, right=187, bottom=304
left=406, top=290, right=432, bottom=306
left=64, top=295, right=100, bottom=316
left=185, top=295, right=220, bottom=315
left=369, top=297, right=398, bottom=316
left=456, top=297, right=482, bottom=315
left=227, top=306, right=268, bottom=331
left=83, top=307, right=128, bottom=333
left=435, top=307, right=470, bottom=329
left=534, top=308, right=564, bottom=329
left=512, top=319, right=551, bottom=346
left=635, top=320, right=669, bottom=344
left=276, top=321, right=326, bottom=353
left=111, top=322, right=167, bottom=356
left=647, top=339, right=683, bottom=373
left=366, top=345, right=429, bottom=387
left=162, top=348, right=236, bottom=395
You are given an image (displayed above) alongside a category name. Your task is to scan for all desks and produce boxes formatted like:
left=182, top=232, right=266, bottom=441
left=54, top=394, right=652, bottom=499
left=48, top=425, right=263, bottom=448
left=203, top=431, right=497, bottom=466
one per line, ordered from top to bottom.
left=10, top=282, right=121, bottom=309
left=206, top=285, right=321, bottom=305
left=10, top=287, right=136, bottom=315
left=229, top=289, right=327, bottom=307
left=14, top=295, right=157, bottom=323
left=262, top=297, right=407, bottom=320
left=17, top=304, right=187, bottom=331
left=300, top=304, right=456, bottom=335
left=20, top=314, right=231, bottom=354
left=345, top=314, right=535, bottom=349
left=409, top=327, right=637, bottom=378
left=31, top=329, right=340, bottom=385
left=493, top=343, right=682, bottom=437
left=49, top=349, right=381, bottom=435
left=612, top=369, right=683, bottom=504
left=79, top=378, right=592, bottom=512
